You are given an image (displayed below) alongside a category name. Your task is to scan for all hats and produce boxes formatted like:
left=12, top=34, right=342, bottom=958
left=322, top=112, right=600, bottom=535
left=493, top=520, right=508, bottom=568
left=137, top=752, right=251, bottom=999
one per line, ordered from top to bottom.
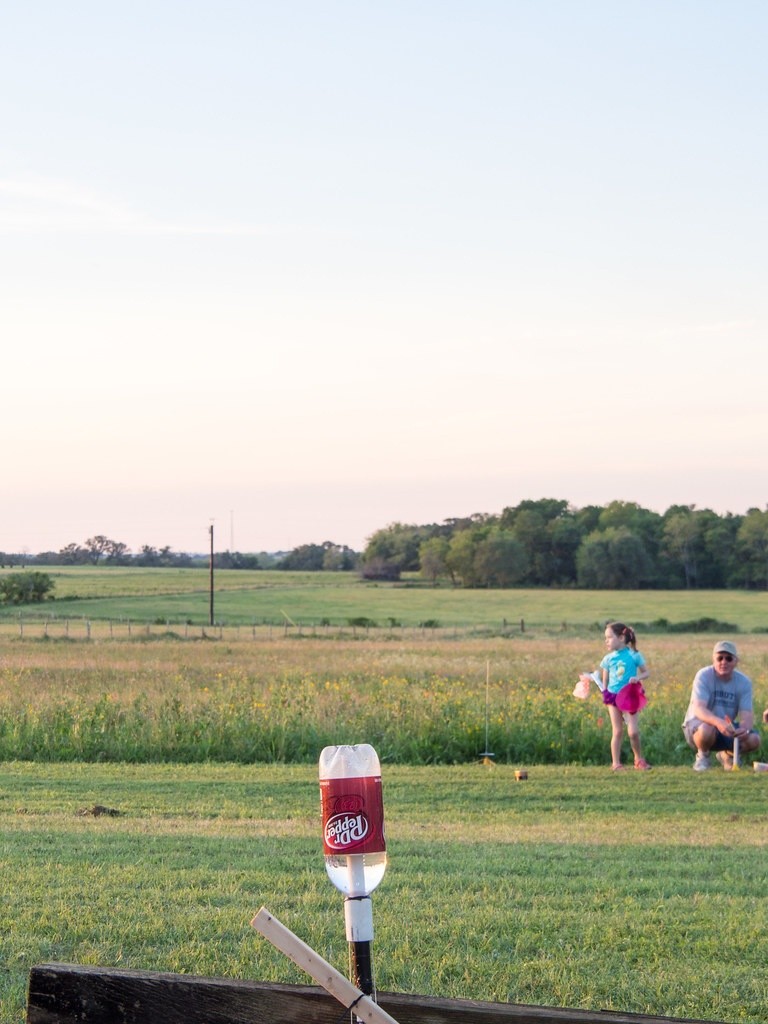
left=713, top=641, right=737, bottom=655
left=614, top=680, right=648, bottom=712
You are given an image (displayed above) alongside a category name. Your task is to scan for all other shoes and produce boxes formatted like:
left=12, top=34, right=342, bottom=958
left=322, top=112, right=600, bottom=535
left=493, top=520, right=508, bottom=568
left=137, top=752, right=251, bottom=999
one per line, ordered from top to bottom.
left=716, top=751, right=734, bottom=772
left=693, top=753, right=708, bottom=772
left=634, top=758, right=651, bottom=770
left=611, top=763, right=623, bottom=770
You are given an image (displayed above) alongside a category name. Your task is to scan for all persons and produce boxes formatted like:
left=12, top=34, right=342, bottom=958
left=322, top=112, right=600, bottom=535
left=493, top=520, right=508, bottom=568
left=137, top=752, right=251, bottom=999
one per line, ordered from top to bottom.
left=579, top=621, right=654, bottom=772
left=681, top=640, right=768, bottom=773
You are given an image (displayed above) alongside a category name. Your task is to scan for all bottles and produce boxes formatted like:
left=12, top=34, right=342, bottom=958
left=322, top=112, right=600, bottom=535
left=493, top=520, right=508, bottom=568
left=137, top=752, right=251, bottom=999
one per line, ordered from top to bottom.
left=319, top=744, right=387, bottom=900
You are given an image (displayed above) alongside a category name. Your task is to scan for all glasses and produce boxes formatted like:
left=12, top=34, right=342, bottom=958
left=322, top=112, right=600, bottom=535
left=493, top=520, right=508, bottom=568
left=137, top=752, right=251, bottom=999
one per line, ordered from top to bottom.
left=714, top=656, right=736, bottom=662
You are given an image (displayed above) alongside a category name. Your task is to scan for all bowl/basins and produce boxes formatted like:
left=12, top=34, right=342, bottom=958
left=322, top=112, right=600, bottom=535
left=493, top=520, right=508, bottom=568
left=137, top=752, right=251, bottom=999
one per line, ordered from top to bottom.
left=753, top=761, right=768, bottom=773
